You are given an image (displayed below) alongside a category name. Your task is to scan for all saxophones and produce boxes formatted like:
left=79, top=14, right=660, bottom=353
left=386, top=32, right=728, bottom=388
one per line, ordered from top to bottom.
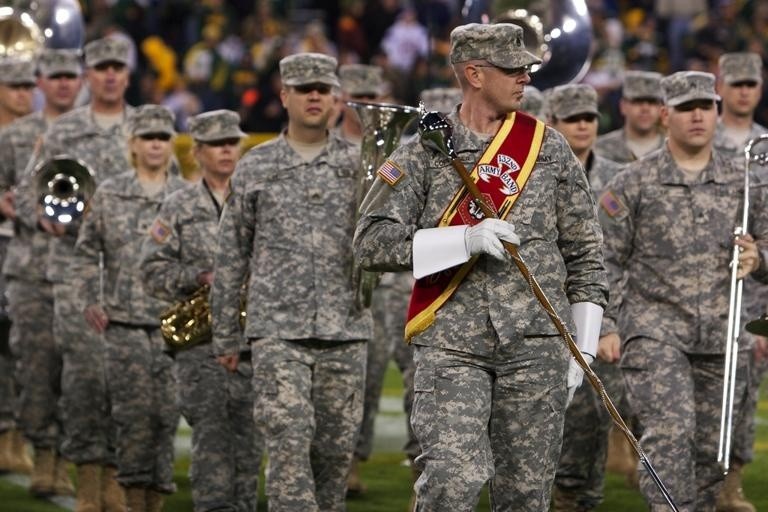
left=346, top=98, right=419, bottom=318
left=34, top=158, right=96, bottom=221
left=158, top=284, right=246, bottom=348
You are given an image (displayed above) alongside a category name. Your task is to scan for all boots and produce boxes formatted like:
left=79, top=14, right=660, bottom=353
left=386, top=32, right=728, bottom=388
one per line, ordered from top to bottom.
left=610, top=418, right=637, bottom=472
left=2, top=430, right=164, bottom=511
left=346, top=457, right=365, bottom=495
left=717, top=464, right=756, bottom=511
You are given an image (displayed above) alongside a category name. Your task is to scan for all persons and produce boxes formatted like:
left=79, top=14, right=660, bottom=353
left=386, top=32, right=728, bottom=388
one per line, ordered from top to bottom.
left=0, top=0, right=767, bottom=512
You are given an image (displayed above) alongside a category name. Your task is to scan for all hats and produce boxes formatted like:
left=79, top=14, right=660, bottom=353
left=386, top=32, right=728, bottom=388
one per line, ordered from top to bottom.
left=450, top=22, right=543, bottom=69
left=0, top=35, right=138, bottom=87
left=279, top=52, right=385, bottom=98
left=718, top=53, right=763, bottom=85
left=621, top=71, right=721, bottom=105
left=421, top=83, right=602, bottom=119
left=123, top=103, right=178, bottom=140
left=191, top=108, right=249, bottom=141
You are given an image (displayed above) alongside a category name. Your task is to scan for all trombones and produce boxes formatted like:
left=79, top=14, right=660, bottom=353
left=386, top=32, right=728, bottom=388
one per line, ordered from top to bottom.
left=714, top=135, right=767, bottom=475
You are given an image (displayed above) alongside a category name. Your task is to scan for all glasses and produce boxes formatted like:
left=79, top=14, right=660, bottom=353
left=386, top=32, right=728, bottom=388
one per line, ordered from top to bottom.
left=470, top=62, right=528, bottom=77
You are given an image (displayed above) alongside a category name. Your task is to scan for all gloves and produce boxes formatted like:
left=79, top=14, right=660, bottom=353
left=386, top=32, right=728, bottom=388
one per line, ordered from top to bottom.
left=413, top=218, right=521, bottom=280
left=565, top=302, right=606, bottom=409
